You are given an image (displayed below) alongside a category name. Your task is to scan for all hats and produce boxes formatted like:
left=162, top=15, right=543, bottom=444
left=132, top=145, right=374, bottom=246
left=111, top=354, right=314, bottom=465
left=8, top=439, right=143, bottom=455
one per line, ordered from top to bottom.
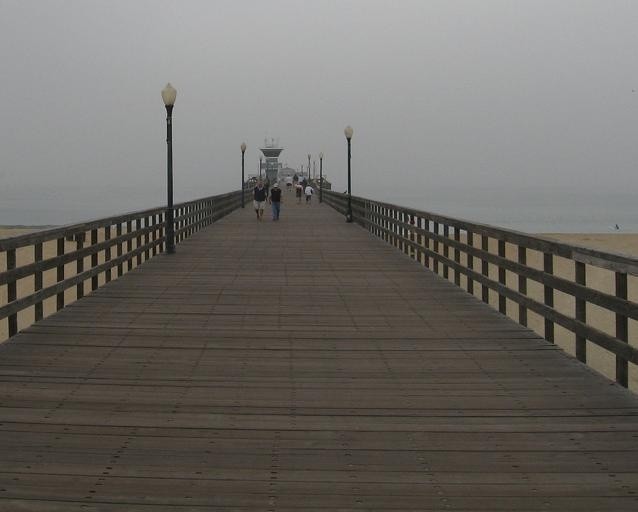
left=273, top=183, right=279, bottom=188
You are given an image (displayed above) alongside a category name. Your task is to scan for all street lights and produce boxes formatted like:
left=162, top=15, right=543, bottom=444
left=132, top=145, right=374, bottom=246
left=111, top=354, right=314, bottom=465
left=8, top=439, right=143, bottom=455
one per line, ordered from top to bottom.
left=160, top=80, right=179, bottom=253
left=344, top=124, right=354, bottom=221
left=240, top=140, right=247, bottom=209
left=306, top=152, right=311, bottom=180
left=319, top=152, right=324, bottom=203
left=257, top=153, right=262, bottom=182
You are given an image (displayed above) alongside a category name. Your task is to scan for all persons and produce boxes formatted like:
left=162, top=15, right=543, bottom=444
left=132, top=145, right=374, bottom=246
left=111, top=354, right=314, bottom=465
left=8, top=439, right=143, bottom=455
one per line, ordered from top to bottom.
left=284, top=174, right=292, bottom=191
left=292, top=174, right=298, bottom=186
left=298, top=174, right=304, bottom=185
left=252, top=181, right=268, bottom=220
left=268, top=183, right=284, bottom=221
left=294, top=183, right=304, bottom=205
left=304, top=184, right=315, bottom=208
